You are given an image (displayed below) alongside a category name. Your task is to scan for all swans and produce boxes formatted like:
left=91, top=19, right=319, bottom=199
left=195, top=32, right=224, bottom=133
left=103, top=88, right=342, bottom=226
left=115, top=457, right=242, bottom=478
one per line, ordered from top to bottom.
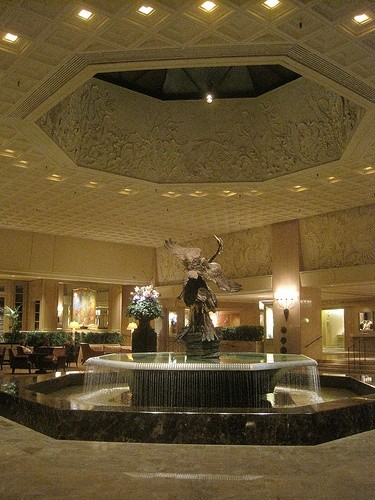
left=162, top=232, right=245, bottom=344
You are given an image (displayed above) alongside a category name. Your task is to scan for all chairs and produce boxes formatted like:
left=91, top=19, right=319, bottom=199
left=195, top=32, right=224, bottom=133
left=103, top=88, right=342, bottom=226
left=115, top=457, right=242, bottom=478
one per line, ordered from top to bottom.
left=81, top=343, right=113, bottom=365
left=0, top=345, right=78, bottom=374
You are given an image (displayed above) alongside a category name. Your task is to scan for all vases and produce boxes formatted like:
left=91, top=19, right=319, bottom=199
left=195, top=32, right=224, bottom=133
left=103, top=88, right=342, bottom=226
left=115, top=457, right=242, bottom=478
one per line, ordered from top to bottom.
left=132, top=321, right=157, bottom=353
left=216, top=325, right=264, bottom=354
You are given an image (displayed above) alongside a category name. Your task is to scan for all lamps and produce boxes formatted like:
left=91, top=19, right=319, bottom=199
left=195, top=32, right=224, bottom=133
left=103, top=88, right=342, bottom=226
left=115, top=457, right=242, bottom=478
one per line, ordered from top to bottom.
left=273, top=284, right=299, bottom=323
left=68, top=320, right=80, bottom=347
left=127, top=321, right=138, bottom=346
left=88, top=323, right=98, bottom=330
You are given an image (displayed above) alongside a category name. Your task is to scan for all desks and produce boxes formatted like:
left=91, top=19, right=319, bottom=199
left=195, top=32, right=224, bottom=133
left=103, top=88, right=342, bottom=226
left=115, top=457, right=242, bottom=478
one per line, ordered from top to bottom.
left=36, top=347, right=64, bottom=357
left=28, top=353, right=55, bottom=374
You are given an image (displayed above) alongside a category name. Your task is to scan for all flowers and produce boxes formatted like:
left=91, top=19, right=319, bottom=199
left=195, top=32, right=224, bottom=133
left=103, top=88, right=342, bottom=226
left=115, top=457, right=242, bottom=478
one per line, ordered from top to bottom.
left=125, top=285, right=165, bottom=321
